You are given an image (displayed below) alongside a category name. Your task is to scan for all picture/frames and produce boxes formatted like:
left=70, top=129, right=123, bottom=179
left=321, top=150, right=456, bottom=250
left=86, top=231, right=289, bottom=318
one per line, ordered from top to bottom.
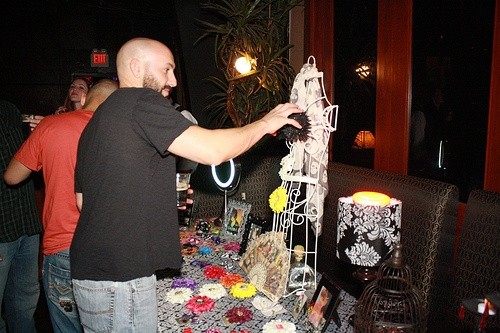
left=222, top=199, right=250, bottom=244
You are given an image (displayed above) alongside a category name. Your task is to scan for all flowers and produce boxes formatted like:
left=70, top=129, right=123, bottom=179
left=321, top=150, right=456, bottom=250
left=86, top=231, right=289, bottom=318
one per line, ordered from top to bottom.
left=268, top=186, right=288, bottom=213
left=203, top=266, right=257, bottom=298
left=165, top=278, right=228, bottom=312
left=225, top=306, right=253, bottom=323
left=262, top=319, right=296, bottom=333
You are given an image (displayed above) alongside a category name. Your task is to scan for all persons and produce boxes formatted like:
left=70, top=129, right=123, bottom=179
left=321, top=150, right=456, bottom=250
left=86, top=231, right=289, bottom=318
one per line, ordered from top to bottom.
left=69, top=37, right=304, bottom=333
left=0, top=76, right=119, bottom=333
left=412, top=88, right=453, bottom=174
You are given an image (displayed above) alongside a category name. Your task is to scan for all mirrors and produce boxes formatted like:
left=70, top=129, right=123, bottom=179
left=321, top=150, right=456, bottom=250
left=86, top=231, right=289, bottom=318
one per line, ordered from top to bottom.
left=211, top=159, right=241, bottom=227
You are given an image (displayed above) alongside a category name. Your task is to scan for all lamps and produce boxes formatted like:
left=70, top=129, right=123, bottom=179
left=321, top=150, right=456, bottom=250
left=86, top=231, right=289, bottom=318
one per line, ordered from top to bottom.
left=352, top=131, right=376, bottom=168
left=335, top=191, right=402, bottom=328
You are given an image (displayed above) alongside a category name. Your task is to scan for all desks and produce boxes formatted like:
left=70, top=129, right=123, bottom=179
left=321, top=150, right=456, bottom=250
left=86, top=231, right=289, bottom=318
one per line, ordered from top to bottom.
left=157, top=224, right=359, bottom=333
